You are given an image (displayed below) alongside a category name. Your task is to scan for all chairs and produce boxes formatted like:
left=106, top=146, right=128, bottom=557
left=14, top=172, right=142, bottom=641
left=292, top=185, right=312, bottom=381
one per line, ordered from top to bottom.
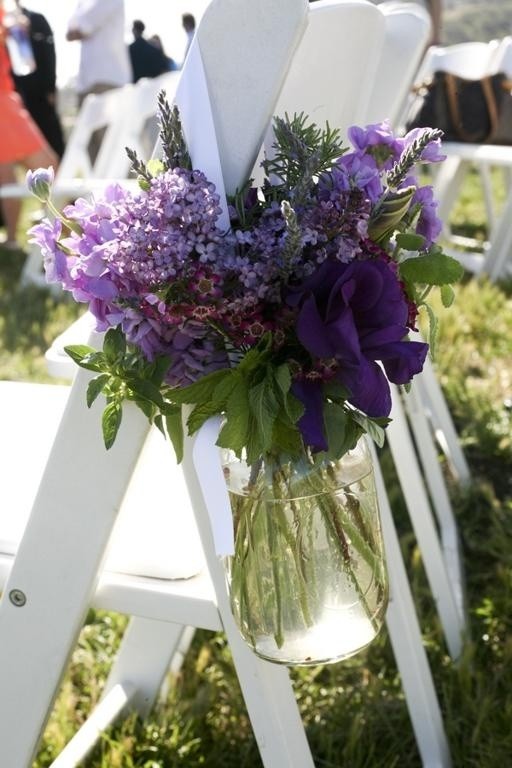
left=0, top=0, right=512, bottom=768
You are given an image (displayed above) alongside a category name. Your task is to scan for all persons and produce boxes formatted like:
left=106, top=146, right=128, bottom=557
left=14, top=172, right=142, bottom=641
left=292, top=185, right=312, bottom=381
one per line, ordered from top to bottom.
left=1, top=1, right=65, bottom=253
left=66, top=1, right=197, bottom=164
